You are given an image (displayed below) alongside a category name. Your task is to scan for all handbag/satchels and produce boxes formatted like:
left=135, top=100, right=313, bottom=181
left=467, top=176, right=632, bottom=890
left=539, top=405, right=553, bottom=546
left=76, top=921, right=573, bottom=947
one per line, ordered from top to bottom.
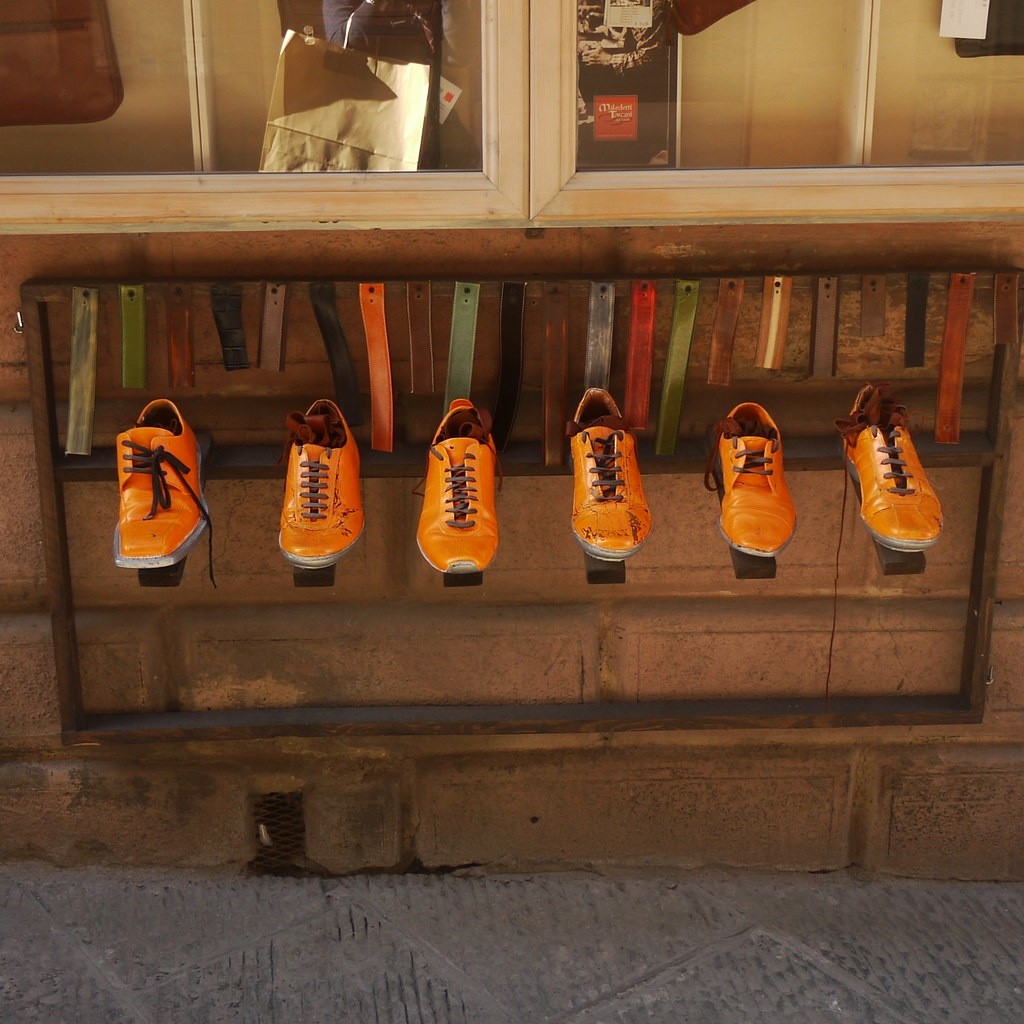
left=259, top=10, right=433, bottom=173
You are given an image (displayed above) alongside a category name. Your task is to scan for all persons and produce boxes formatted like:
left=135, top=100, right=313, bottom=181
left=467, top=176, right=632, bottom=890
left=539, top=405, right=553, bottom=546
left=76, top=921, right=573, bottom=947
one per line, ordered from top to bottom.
left=323, top=0, right=443, bottom=169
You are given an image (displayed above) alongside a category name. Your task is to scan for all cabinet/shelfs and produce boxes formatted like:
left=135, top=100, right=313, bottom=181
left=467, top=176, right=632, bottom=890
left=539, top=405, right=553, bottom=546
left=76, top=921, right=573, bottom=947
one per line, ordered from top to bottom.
left=0, top=0, right=1023, bottom=234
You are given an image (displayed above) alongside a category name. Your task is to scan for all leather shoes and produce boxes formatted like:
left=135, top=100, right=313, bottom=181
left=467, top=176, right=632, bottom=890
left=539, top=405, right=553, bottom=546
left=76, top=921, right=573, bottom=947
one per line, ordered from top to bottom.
left=833, top=382, right=944, bottom=552
left=565, top=388, right=653, bottom=562
left=411, top=398, right=503, bottom=573
left=114, top=399, right=217, bottom=588
left=279, top=399, right=366, bottom=569
left=704, top=402, right=797, bottom=557
